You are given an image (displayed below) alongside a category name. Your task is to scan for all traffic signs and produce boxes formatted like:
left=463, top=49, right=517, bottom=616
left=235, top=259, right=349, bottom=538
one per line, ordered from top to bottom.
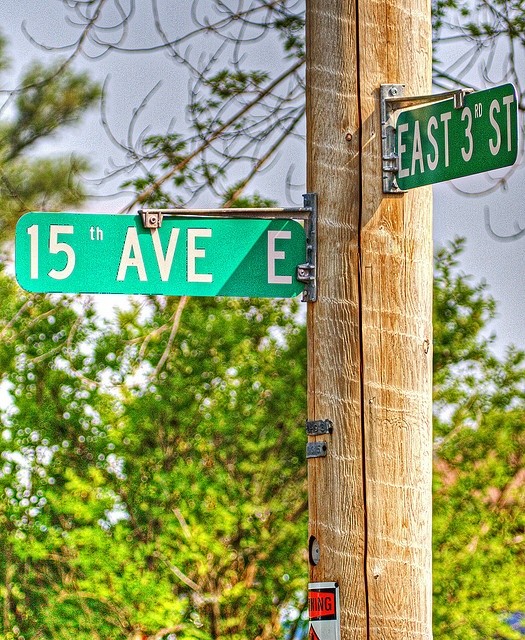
left=395, top=84, right=518, bottom=191
left=13, top=211, right=308, bottom=298
